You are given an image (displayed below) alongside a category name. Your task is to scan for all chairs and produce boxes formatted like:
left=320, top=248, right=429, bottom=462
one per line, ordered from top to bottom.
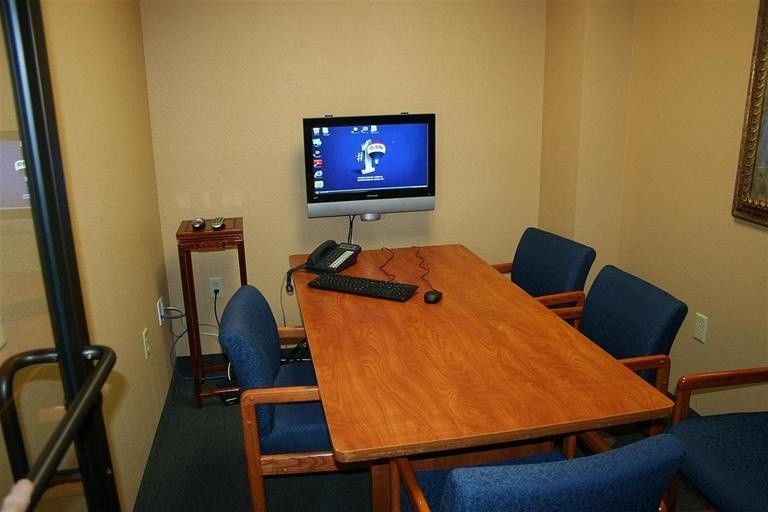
left=488, top=226, right=597, bottom=330
left=547, top=263, right=689, bottom=438
left=216, top=283, right=390, bottom=511
left=662, top=367, right=767, bottom=512
left=386, top=426, right=687, bottom=512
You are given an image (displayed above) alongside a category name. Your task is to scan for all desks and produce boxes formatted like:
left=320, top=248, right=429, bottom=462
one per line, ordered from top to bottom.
left=175, top=216, right=248, bottom=411
left=287, top=243, right=674, bottom=512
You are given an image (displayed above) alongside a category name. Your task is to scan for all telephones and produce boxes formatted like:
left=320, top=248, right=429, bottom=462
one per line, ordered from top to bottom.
left=305, top=240, right=361, bottom=273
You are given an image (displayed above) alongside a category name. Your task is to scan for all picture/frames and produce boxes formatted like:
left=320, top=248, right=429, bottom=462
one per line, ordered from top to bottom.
left=727, top=0, right=767, bottom=231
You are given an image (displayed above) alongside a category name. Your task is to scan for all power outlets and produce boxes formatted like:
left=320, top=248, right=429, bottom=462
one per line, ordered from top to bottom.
left=206, top=276, right=223, bottom=299
left=155, top=294, right=167, bottom=327
left=692, top=309, right=708, bottom=344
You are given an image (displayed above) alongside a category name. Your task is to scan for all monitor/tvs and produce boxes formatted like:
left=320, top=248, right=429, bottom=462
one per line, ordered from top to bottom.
left=303, top=113, right=436, bottom=218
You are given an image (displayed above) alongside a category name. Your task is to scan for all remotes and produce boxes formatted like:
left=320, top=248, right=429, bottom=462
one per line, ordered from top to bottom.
left=211, top=216, right=225, bottom=229
left=192, top=217, right=204, bottom=229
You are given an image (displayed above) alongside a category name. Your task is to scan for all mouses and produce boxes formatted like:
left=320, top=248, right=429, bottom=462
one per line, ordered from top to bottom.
left=423, top=289, right=442, bottom=304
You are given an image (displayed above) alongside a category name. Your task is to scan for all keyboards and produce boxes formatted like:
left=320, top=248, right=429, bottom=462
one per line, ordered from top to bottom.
left=306, top=273, right=419, bottom=302
